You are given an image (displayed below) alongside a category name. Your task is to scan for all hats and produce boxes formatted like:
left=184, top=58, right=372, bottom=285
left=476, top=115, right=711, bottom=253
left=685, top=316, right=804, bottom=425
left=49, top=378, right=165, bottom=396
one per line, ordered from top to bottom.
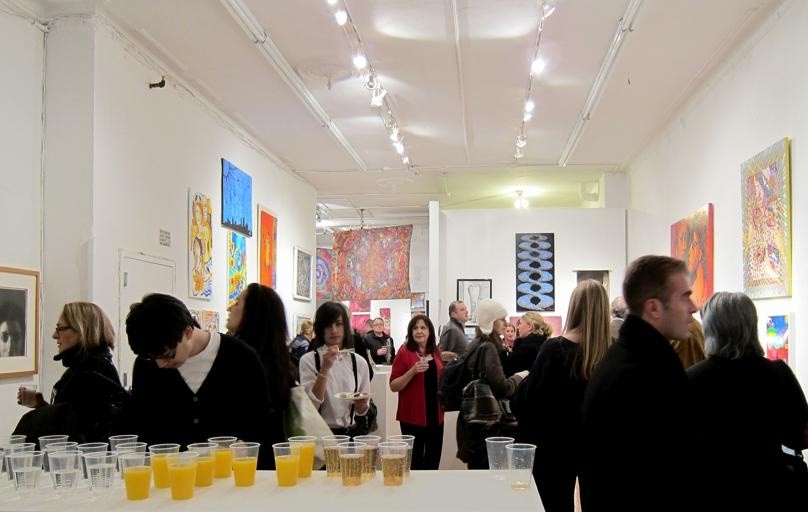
left=476, top=298, right=507, bottom=337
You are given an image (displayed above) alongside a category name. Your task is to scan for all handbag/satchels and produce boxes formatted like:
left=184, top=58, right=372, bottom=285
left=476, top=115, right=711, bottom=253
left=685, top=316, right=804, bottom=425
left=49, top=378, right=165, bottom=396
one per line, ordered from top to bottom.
left=438, top=357, right=483, bottom=411
left=460, top=371, right=518, bottom=430
left=282, top=385, right=338, bottom=470
left=354, top=401, right=377, bottom=433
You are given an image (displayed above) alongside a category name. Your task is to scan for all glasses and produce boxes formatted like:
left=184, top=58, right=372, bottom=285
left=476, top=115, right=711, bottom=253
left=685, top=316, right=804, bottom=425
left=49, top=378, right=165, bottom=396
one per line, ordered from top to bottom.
left=373, top=322, right=384, bottom=327
left=56, top=327, right=71, bottom=334
left=1, top=333, right=10, bottom=342
left=141, top=349, right=176, bottom=362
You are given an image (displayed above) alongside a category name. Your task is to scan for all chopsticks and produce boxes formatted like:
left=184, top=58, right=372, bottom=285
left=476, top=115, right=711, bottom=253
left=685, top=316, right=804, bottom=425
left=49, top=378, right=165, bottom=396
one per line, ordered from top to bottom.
left=318, top=348, right=356, bottom=355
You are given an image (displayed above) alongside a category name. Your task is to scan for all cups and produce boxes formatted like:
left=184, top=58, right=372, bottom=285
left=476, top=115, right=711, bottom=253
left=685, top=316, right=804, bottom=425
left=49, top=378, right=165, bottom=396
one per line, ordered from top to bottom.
left=381, top=345, right=387, bottom=355
left=418, top=356, right=430, bottom=372
left=19, top=384, right=38, bottom=407
left=0, top=433, right=28, bottom=453
left=38, top=434, right=69, bottom=473
left=108, top=434, right=140, bottom=473
left=320, top=434, right=351, bottom=480
left=385, top=434, right=416, bottom=479
left=207, top=435, right=238, bottom=480
left=287, top=435, right=318, bottom=480
left=352, top=435, right=382, bottom=480
left=484, top=436, right=516, bottom=481
left=44, top=441, right=78, bottom=453
left=76, top=441, right=109, bottom=480
left=228, top=441, right=261, bottom=489
left=336, top=441, right=368, bottom=487
left=3, top=442, right=36, bottom=482
left=114, top=442, right=148, bottom=481
left=186, top=442, right=220, bottom=489
left=271, top=442, right=303, bottom=488
left=377, top=442, right=409, bottom=488
left=147, top=443, right=182, bottom=489
left=504, top=443, right=538, bottom=492
left=10, top=450, right=44, bottom=493
left=47, top=450, right=84, bottom=496
left=82, top=450, right=118, bottom=496
left=0, top=451, right=6, bottom=474
left=165, top=451, right=200, bottom=500
left=121, top=452, right=155, bottom=502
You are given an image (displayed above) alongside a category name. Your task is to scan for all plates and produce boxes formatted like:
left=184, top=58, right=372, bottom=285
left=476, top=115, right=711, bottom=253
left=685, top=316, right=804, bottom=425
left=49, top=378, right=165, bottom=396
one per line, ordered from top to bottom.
left=334, top=392, right=368, bottom=400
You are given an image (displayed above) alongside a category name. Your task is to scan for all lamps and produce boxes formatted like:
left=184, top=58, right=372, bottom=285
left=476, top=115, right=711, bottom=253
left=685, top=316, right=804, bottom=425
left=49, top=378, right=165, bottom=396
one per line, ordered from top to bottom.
left=538, top=0, right=556, bottom=17
left=514, top=190, right=528, bottom=208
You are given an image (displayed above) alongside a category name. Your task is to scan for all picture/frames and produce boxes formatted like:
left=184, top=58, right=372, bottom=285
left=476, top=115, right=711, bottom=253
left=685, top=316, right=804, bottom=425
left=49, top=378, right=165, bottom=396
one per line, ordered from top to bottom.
left=258, top=204, right=278, bottom=291
left=293, top=244, right=313, bottom=302
left=1, top=268, right=39, bottom=379
left=457, top=278, right=492, bottom=328
left=765, top=312, right=795, bottom=371
left=294, top=313, right=313, bottom=338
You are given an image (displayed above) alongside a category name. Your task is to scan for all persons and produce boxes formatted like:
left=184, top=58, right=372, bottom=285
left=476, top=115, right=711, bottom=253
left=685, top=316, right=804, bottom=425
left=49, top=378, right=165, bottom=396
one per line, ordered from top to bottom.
left=190, top=193, right=211, bottom=290
left=676, top=220, right=690, bottom=264
left=685, top=227, right=709, bottom=309
left=584, top=254, right=723, bottom=511
left=510, top=278, right=617, bottom=512
left=225, top=283, right=292, bottom=421
left=465, top=283, right=483, bottom=322
left=125, top=291, right=285, bottom=471
left=683, top=291, right=808, bottom=511
left=608, top=295, right=633, bottom=345
left=286, top=298, right=552, bottom=471
left=0, top=299, right=25, bottom=356
left=12, top=302, right=131, bottom=461
left=669, top=316, right=704, bottom=370
